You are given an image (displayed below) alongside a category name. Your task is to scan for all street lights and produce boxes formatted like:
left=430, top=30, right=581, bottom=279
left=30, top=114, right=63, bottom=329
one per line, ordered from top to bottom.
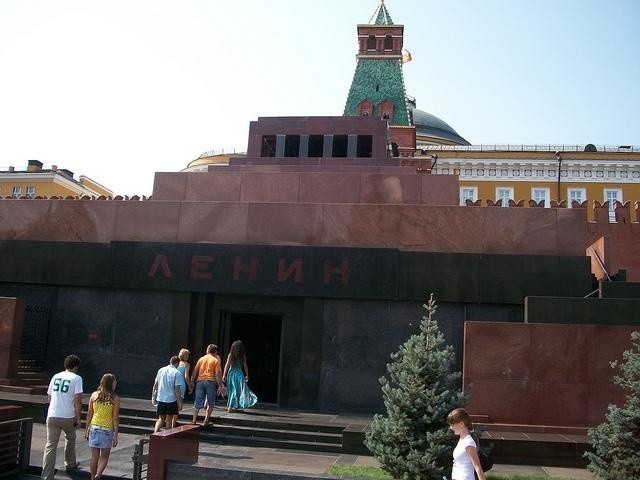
left=555, top=150, right=563, bottom=203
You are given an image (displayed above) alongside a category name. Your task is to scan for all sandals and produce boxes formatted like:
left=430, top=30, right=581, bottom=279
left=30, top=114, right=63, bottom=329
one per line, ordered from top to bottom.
left=191, top=408, right=236, bottom=425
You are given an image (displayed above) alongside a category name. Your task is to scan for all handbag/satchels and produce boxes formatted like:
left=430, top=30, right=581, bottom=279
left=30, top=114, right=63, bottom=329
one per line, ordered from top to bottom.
left=474, top=450, right=494, bottom=479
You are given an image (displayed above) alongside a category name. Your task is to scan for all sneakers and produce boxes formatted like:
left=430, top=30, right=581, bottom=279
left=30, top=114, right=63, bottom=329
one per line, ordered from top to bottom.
left=65, top=462, right=80, bottom=472
left=93, top=474, right=100, bottom=480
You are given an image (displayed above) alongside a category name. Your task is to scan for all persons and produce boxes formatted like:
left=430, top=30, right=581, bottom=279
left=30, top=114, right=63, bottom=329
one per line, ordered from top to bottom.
left=221, top=339, right=260, bottom=413
left=190, top=342, right=222, bottom=429
left=172, top=347, right=192, bottom=428
left=38, top=352, right=85, bottom=480
left=150, top=354, right=183, bottom=434
left=203, top=355, right=222, bottom=411
left=84, top=371, right=122, bottom=480
left=446, top=407, right=486, bottom=480
